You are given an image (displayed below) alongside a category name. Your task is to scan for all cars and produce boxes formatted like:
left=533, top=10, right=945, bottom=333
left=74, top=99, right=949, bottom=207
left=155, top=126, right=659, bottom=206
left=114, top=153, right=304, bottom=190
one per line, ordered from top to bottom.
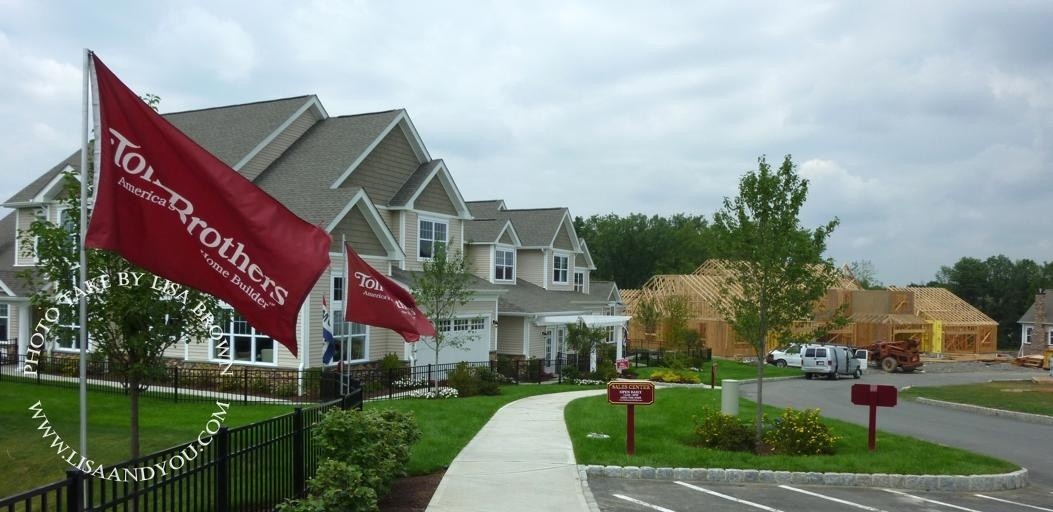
left=850, top=345, right=868, bottom=370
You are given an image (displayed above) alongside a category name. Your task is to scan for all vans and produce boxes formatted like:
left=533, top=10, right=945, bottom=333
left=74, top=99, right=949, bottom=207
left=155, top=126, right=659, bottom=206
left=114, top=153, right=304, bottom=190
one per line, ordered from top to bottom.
left=764, top=341, right=822, bottom=370
left=800, top=345, right=863, bottom=381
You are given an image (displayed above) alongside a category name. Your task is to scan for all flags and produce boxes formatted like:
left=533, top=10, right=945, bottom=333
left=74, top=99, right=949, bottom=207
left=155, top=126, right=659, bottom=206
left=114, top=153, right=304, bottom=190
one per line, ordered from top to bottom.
left=84, top=51, right=333, bottom=358
left=343, top=240, right=439, bottom=343
left=321, top=296, right=336, bottom=365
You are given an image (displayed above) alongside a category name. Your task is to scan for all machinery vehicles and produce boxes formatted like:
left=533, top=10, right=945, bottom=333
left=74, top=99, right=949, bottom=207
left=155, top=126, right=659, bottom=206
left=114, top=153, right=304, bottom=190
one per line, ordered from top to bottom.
left=857, top=335, right=926, bottom=373
